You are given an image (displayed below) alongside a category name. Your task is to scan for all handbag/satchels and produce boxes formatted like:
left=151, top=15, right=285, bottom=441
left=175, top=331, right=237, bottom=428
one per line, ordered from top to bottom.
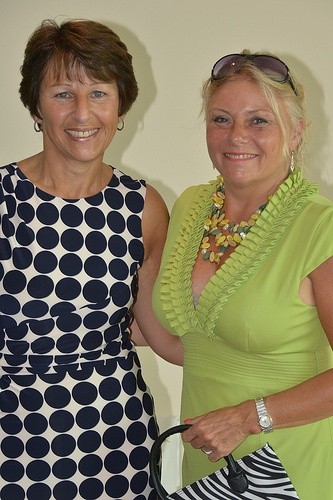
left=150, top=424, right=300, bottom=500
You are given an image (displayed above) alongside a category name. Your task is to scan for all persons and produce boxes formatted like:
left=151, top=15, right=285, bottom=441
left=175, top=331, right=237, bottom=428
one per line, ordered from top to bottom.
left=0, top=17, right=182, bottom=500
left=131, top=48, right=330, bottom=500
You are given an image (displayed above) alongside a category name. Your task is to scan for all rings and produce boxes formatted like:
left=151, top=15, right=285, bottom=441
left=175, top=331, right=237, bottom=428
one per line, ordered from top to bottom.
left=201, top=447, right=213, bottom=456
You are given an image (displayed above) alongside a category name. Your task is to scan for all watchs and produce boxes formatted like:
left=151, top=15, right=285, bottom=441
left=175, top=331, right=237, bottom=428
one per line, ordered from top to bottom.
left=254, top=397, right=274, bottom=435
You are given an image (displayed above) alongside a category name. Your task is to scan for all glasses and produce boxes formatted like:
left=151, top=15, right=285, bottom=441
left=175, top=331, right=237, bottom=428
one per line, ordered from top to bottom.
left=210, top=52, right=298, bottom=96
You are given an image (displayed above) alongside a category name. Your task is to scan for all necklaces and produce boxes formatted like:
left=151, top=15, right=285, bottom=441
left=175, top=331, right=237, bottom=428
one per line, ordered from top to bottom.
left=199, top=173, right=272, bottom=268
